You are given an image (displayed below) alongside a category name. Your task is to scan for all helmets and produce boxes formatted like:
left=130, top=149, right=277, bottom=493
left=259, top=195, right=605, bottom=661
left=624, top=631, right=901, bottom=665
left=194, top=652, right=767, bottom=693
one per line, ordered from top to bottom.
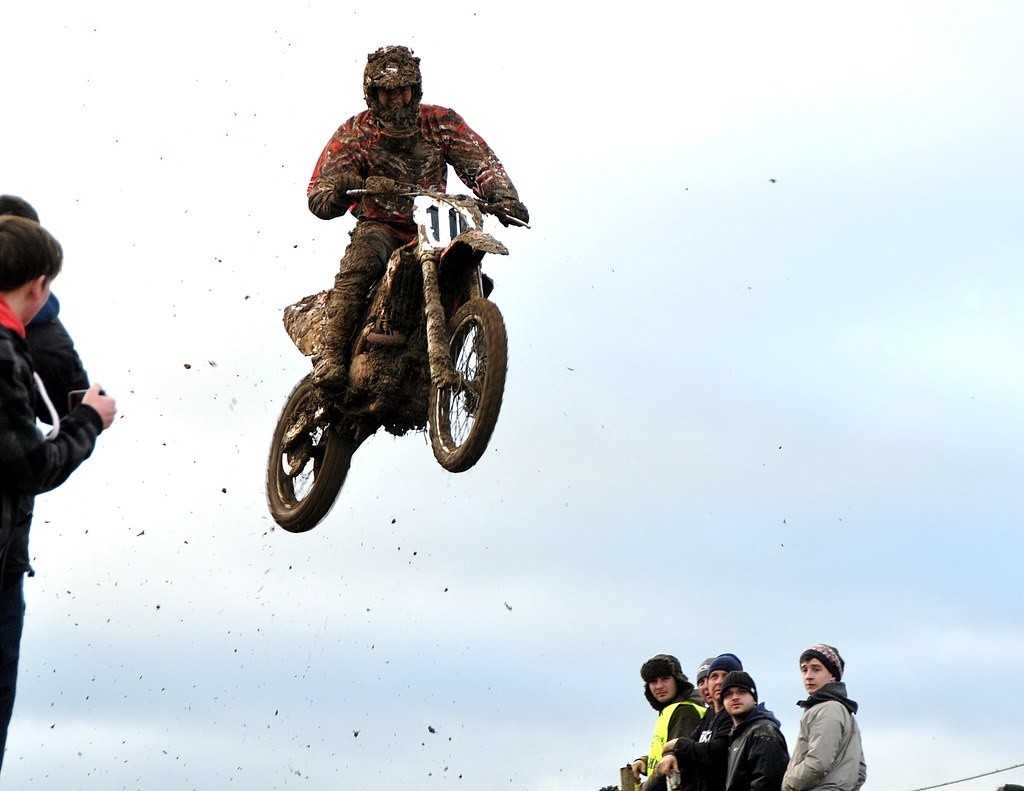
left=362, top=45, right=424, bottom=136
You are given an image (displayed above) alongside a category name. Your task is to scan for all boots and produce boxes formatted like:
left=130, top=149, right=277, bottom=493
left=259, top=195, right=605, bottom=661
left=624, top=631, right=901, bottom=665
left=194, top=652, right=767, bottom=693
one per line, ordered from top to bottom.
left=311, top=299, right=370, bottom=389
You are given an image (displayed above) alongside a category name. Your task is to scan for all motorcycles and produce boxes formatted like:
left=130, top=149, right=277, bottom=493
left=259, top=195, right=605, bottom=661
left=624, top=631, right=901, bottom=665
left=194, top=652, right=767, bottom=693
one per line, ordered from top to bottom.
left=266, top=177, right=531, bottom=533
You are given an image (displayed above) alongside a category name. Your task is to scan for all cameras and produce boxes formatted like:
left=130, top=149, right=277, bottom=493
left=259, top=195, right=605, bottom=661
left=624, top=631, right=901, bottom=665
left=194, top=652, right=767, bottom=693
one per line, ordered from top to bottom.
left=69, top=390, right=106, bottom=412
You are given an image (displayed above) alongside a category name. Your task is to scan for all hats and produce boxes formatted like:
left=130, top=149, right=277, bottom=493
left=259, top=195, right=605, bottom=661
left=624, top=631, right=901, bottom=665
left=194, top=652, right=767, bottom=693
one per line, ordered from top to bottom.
left=799, top=644, right=845, bottom=682
left=707, top=653, right=743, bottom=679
left=640, top=655, right=694, bottom=712
left=696, top=658, right=716, bottom=684
left=719, top=671, right=757, bottom=704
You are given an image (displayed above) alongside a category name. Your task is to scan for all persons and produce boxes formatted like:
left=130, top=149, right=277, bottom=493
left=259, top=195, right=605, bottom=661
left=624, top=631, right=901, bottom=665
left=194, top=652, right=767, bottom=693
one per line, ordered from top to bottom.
left=308, top=47, right=529, bottom=385
left=0, top=196, right=117, bottom=770
left=782, top=645, right=867, bottom=791
left=657, top=653, right=743, bottom=791
left=632, top=654, right=707, bottom=785
left=720, top=670, right=790, bottom=791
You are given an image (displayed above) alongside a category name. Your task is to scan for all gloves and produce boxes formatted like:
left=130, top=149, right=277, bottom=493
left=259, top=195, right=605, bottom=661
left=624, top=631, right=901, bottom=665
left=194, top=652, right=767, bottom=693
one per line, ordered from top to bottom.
left=329, top=171, right=366, bottom=207
left=493, top=197, right=529, bottom=227
